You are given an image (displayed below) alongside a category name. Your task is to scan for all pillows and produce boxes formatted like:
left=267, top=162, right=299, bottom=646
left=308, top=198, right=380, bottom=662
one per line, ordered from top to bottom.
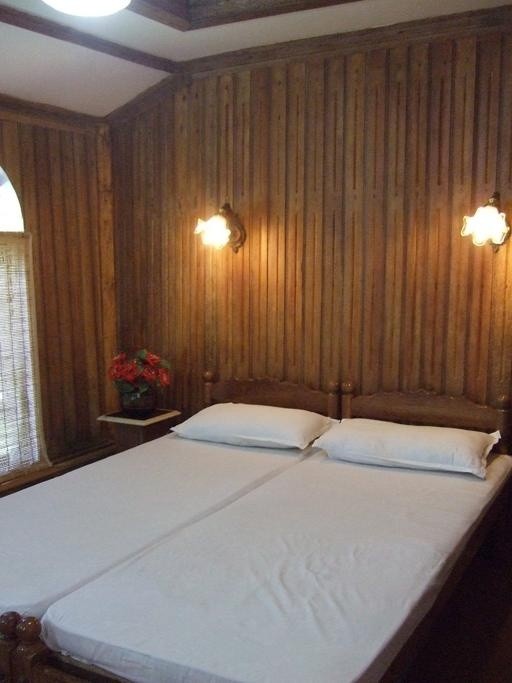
left=169, top=402, right=338, bottom=449
left=312, top=418, right=501, bottom=479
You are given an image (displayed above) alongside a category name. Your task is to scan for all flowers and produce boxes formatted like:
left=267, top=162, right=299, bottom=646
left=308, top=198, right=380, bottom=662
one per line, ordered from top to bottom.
left=105, top=347, right=177, bottom=394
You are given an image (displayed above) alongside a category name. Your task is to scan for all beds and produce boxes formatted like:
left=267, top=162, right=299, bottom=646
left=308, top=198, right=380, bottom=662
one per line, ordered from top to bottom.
left=1, top=371, right=338, bottom=682
left=11, top=381, right=511, bottom=683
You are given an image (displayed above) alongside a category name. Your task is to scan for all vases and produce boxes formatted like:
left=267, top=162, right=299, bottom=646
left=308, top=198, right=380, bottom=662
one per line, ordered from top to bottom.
left=96, top=408, right=181, bottom=451
